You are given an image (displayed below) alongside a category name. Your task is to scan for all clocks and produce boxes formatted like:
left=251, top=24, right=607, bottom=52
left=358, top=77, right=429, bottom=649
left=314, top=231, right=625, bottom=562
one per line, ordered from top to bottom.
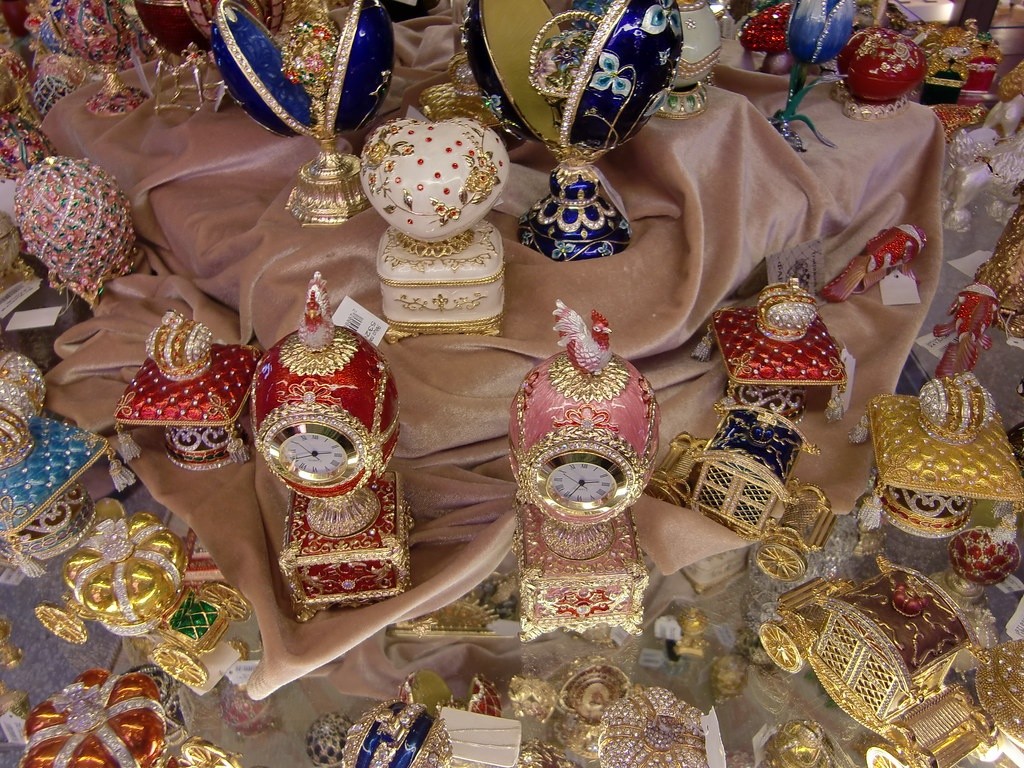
left=245, top=268, right=414, bottom=619
left=508, top=299, right=660, bottom=643
